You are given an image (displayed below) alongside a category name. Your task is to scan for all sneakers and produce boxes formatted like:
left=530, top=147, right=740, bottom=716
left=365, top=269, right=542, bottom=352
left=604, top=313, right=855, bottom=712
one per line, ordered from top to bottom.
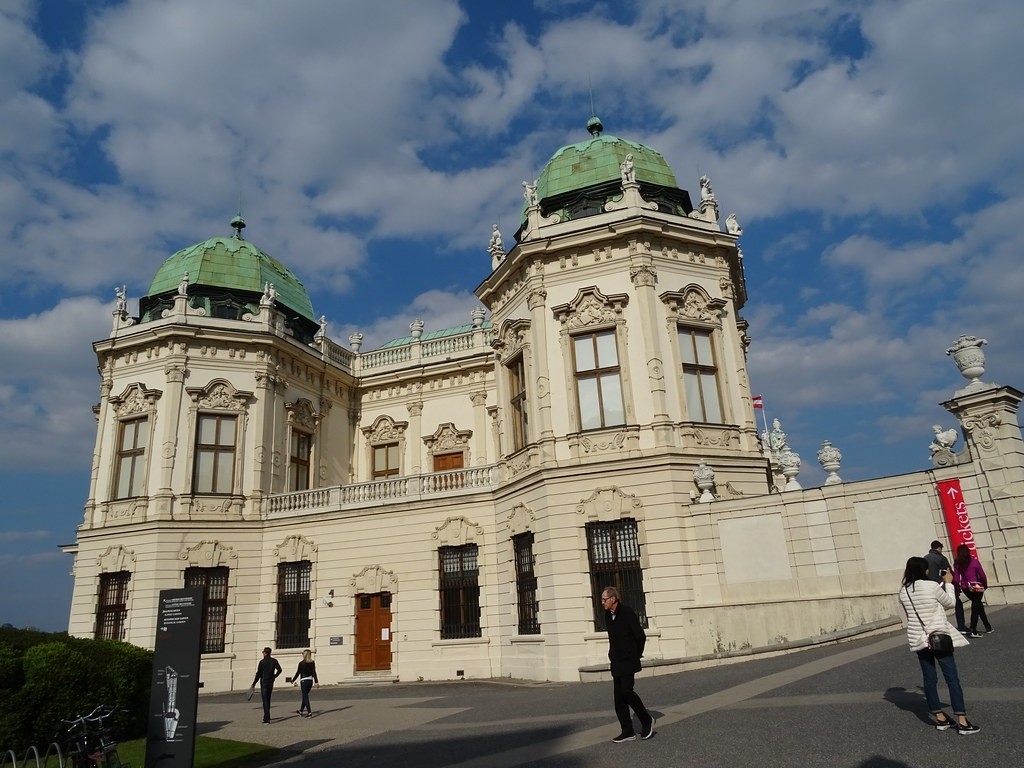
left=958, top=627, right=970, bottom=634
left=987, top=628, right=994, bottom=633
left=970, top=632, right=983, bottom=638
left=641, top=716, right=656, bottom=739
left=959, top=717, right=980, bottom=735
left=937, top=718, right=958, bottom=730
left=612, top=732, right=637, bottom=742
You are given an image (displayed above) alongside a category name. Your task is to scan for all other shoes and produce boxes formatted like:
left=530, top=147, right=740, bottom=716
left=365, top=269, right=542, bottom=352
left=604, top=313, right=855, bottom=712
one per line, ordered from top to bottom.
left=296, top=710, right=303, bottom=717
left=305, top=713, right=312, bottom=718
left=262, top=722, right=270, bottom=724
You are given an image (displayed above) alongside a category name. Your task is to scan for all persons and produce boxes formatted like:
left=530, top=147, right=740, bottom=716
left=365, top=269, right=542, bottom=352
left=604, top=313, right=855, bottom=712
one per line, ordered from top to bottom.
left=620, top=154, right=635, bottom=182
left=699, top=176, right=712, bottom=193
left=522, top=181, right=540, bottom=207
left=728, top=214, right=742, bottom=232
left=488, top=224, right=503, bottom=250
left=182, top=271, right=189, bottom=294
left=264, top=283, right=280, bottom=302
left=115, top=287, right=127, bottom=310
left=319, top=315, right=327, bottom=334
left=929, top=425, right=947, bottom=460
left=924, top=540, right=970, bottom=634
left=954, top=544, right=994, bottom=637
left=898, top=557, right=980, bottom=734
left=602, top=587, right=655, bottom=743
left=251, top=647, right=282, bottom=724
left=291, top=649, right=319, bottom=718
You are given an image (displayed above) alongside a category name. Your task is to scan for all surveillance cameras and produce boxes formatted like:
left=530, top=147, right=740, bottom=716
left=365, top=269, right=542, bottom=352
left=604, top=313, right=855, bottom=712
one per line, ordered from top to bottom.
left=689, top=490, right=696, bottom=500
left=323, top=597, right=332, bottom=606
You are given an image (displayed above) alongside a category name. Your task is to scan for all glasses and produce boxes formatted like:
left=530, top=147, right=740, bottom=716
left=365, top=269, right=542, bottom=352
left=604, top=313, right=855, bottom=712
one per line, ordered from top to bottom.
left=600, top=595, right=613, bottom=603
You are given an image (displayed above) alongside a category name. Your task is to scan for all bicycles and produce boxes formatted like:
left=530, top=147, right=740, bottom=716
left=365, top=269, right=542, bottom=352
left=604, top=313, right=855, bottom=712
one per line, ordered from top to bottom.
left=59, top=704, right=129, bottom=768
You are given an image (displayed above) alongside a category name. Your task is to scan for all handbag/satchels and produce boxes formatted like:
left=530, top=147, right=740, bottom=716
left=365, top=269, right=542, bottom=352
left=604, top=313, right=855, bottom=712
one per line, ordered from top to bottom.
left=968, top=581, right=985, bottom=592
left=929, top=634, right=954, bottom=655
left=247, top=688, right=254, bottom=701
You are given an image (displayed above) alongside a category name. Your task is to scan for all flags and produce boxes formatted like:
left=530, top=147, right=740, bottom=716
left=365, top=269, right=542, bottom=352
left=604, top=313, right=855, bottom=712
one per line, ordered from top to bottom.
left=752, top=396, right=762, bottom=408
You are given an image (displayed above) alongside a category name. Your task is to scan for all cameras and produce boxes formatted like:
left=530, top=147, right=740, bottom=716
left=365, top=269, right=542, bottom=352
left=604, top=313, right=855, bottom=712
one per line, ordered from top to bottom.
left=938, top=570, right=946, bottom=577
